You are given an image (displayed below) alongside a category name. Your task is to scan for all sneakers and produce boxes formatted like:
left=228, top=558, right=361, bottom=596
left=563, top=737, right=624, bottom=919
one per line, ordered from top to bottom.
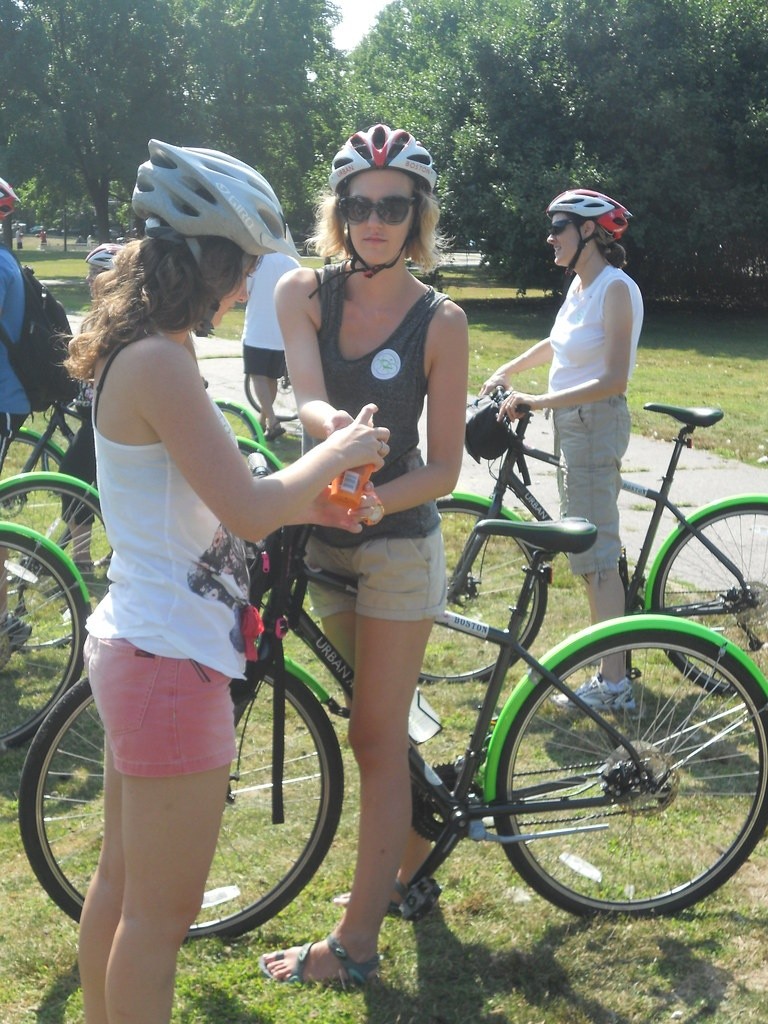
left=554, top=675, right=634, bottom=710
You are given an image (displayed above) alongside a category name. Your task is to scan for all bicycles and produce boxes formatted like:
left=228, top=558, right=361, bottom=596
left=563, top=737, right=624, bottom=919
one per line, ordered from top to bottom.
left=0, top=368, right=290, bottom=769
left=392, top=385, right=768, bottom=699
left=4, top=433, right=767, bottom=945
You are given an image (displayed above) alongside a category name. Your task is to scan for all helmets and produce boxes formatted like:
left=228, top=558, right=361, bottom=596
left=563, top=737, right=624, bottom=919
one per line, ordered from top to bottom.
left=330, top=124, right=438, bottom=194
left=132, top=138, right=302, bottom=262
left=0, top=178, right=21, bottom=220
left=545, top=189, right=634, bottom=240
left=84, top=242, right=124, bottom=271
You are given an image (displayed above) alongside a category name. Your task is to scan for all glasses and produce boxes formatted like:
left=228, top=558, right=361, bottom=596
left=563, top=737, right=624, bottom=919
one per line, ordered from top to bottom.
left=339, top=195, right=419, bottom=226
left=548, top=217, right=576, bottom=235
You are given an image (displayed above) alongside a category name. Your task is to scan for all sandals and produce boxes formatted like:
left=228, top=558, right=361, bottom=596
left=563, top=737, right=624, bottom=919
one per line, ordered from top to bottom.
left=339, top=879, right=407, bottom=913
left=260, top=933, right=381, bottom=986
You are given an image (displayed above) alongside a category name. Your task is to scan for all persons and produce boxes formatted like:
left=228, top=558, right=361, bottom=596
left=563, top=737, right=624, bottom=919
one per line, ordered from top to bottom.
left=257, top=124, right=469, bottom=987
left=65, top=139, right=390, bottom=1024
left=0, top=178, right=33, bottom=669
left=479, top=188, right=644, bottom=710
left=16, top=226, right=22, bottom=250
left=39, top=229, right=47, bottom=244
left=58, top=243, right=122, bottom=576
left=242, top=252, right=301, bottom=442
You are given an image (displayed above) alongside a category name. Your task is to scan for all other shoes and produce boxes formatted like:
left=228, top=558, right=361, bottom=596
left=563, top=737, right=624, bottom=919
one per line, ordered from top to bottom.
left=95, top=554, right=113, bottom=568
left=73, top=562, right=96, bottom=577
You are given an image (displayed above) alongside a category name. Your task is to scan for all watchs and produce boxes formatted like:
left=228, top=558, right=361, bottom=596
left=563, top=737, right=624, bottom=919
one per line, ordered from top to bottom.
left=363, top=492, right=384, bottom=527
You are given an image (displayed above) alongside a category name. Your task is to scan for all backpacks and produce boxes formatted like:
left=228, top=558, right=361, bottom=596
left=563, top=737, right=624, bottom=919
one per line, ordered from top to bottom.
left=0, top=243, right=81, bottom=413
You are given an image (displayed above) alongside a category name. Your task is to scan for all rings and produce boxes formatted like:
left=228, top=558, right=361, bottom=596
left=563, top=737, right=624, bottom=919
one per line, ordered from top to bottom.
left=507, top=402, right=510, bottom=406
left=377, top=440, right=384, bottom=452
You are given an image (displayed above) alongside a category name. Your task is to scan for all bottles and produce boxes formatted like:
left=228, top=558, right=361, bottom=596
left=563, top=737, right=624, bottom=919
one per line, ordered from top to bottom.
left=328, top=414, right=374, bottom=514
left=407, top=691, right=442, bottom=742
